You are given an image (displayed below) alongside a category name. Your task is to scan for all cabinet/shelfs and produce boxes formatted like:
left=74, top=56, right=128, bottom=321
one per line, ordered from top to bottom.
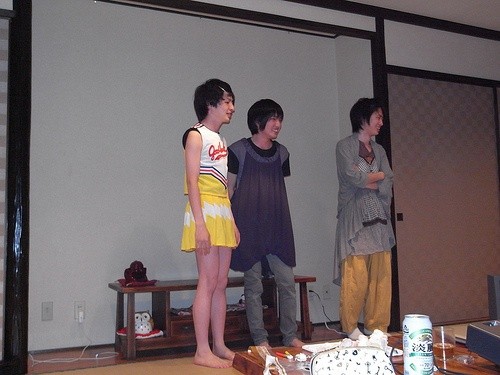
left=109, top=275, right=316, bottom=360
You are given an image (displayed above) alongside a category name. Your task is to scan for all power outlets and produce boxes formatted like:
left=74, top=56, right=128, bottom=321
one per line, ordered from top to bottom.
left=307, top=285, right=315, bottom=299
left=322, top=286, right=330, bottom=300
left=74, top=301, right=85, bottom=321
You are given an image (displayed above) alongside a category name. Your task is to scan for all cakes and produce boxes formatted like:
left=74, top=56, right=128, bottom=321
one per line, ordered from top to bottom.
left=336, top=328, right=388, bottom=353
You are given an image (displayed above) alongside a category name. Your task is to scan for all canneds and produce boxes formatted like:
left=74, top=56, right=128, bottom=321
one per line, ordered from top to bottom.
left=402, top=313, right=434, bottom=375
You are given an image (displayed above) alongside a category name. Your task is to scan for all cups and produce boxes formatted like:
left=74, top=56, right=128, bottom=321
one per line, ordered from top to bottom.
left=433, top=327, right=455, bottom=361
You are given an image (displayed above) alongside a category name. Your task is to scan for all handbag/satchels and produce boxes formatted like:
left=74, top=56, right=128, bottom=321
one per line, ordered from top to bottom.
left=309, top=343, right=395, bottom=375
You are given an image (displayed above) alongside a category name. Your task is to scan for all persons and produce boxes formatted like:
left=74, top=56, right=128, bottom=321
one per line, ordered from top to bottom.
left=183, top=78, right=240, bottom=369
left=227, top=98, right=306, bottom=350
left=332, top=98, right=396, bottom=339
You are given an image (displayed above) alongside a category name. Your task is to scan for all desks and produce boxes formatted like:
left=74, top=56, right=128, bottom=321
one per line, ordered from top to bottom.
left=232, top=331, right=500, bottom=375
